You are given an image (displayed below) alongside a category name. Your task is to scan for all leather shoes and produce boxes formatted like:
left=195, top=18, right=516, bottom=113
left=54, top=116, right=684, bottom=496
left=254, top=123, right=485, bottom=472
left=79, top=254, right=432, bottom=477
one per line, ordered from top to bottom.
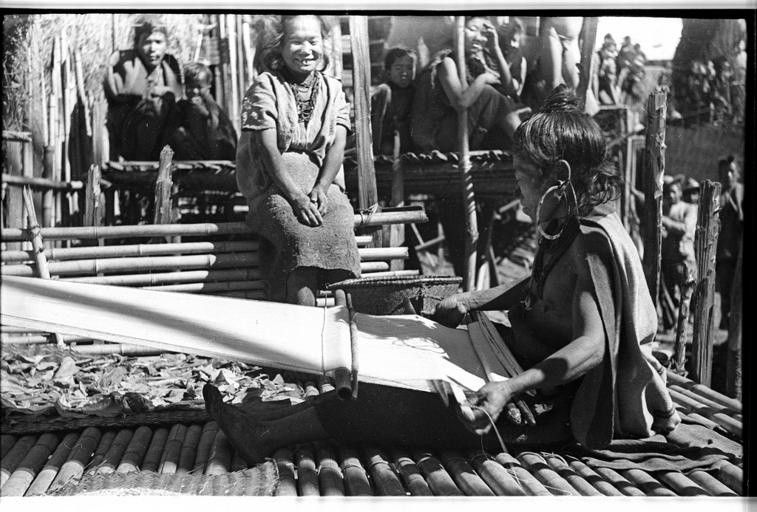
left=203, top=382, right=268, bottom=465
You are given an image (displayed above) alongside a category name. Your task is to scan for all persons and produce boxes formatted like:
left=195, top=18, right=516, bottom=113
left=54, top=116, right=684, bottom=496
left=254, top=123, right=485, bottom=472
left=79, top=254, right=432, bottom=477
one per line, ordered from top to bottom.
left=101, top=12, right=237, bottom=157
left=234, top=12, right=361, bottom=306
left=369, top=13, right=646, bottom=154
left=202, top=84, right=682, bottom=465
left=626, top=152, right=744, bottom=331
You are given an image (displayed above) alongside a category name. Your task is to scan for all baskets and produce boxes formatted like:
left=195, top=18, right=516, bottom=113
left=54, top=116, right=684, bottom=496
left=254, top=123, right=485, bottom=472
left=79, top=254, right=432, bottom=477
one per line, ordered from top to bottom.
left=328, top=274, right=463, bottom=314
